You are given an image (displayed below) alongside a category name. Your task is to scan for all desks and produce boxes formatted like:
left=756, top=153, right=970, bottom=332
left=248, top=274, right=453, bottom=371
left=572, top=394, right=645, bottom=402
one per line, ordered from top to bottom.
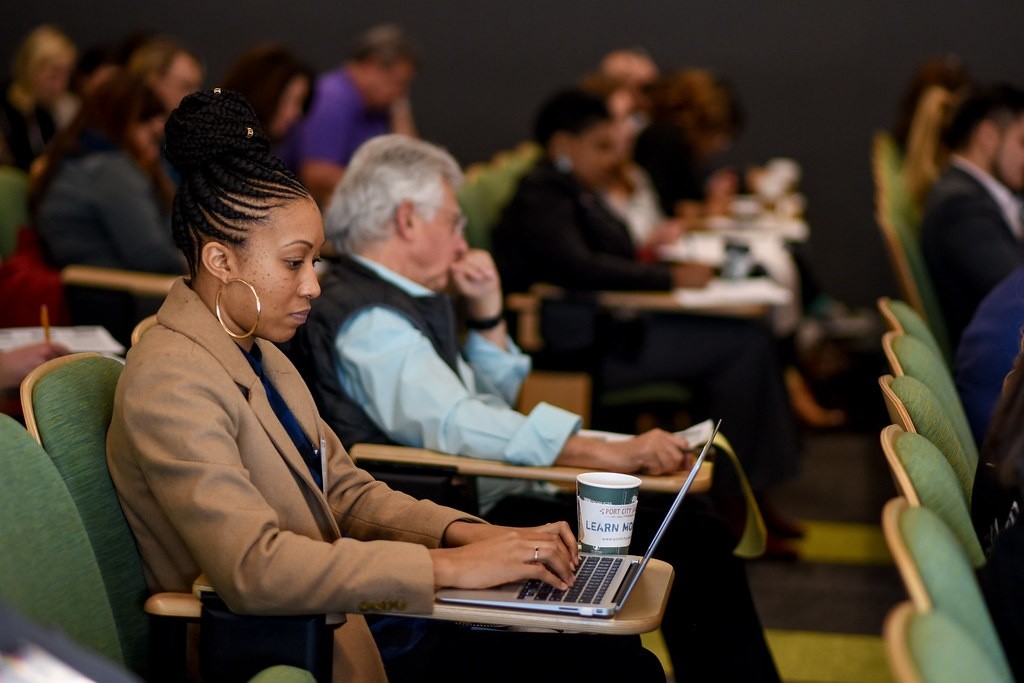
left=458, top=436, right=714, bottom=494
left=395, top=554, right=675, bottom=635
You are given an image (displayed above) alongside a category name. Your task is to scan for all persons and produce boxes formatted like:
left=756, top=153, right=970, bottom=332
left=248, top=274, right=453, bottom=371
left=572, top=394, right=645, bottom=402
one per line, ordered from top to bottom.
left=0, top=24, right=1024, bottom=683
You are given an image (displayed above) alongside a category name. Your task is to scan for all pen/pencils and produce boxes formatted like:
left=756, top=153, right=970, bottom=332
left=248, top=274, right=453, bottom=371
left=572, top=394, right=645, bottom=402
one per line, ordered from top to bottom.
left=40, top=305, right=50, bottom=347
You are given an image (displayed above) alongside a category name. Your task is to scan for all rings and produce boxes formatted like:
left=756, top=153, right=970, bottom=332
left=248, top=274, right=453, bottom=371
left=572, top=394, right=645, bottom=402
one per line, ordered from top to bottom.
left=535, top=545, right=538, bottom=562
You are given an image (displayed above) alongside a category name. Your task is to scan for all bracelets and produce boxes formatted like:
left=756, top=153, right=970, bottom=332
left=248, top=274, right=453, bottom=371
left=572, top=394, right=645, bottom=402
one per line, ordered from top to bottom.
left=465, top=310, right=501, bottom=330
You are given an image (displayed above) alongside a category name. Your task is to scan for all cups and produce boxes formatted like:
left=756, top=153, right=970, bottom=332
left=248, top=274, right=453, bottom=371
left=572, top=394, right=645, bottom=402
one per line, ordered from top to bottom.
left=721, top=233, right=753, bottom=279
left=575, top=472, right=642, bottom=555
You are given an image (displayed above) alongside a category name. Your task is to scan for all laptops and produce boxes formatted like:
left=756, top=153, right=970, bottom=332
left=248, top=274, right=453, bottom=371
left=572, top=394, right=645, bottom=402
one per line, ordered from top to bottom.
left=436, top=418, right=723, bottom=615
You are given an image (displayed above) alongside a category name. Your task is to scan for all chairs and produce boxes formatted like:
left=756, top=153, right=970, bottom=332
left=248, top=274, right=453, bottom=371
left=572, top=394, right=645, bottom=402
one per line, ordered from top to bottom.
left=873, top=136, right=1016, bottom=683
left=460, top=141, right=535, bottom=240
left=1, top=352, right=391, bottom=682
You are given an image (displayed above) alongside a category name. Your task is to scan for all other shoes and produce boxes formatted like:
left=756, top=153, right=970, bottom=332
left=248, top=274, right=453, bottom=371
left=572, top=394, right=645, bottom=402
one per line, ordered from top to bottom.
left=763, top=500, right=803, bottom=540
left=760, top=536, right=800, bottom=567
